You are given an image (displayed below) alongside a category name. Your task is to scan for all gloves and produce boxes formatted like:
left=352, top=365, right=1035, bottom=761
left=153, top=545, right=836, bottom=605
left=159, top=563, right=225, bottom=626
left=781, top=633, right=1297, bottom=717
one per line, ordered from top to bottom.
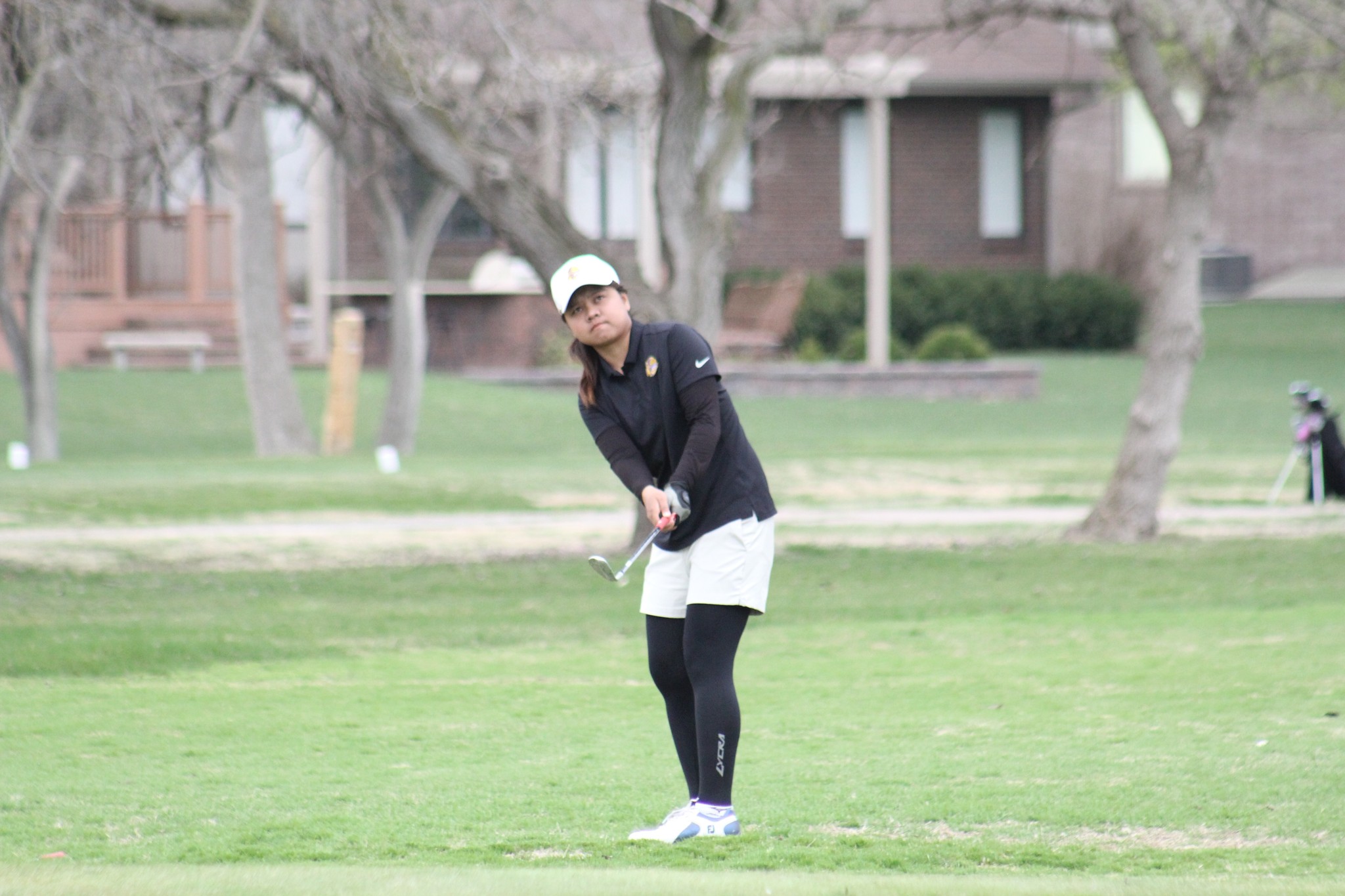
left=659, top=481, right=691, bottom=527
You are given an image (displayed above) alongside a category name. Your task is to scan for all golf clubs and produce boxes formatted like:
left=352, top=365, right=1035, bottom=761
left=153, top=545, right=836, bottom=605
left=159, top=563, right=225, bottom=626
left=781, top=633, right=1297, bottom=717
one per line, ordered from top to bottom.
left=1290, top=389, right=1322, bottom=410
left=587, top=512, right=676, bottom=584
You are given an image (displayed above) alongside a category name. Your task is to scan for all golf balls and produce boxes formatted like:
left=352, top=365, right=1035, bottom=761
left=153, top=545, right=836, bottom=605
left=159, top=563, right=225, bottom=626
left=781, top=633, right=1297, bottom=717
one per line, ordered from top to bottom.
left=375, top=445, right=399, bottom=473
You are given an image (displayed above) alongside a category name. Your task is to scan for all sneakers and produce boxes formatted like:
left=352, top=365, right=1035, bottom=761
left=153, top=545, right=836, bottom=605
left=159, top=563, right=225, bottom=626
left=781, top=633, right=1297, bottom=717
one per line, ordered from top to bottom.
left=628, top=797, right=741, bottom=844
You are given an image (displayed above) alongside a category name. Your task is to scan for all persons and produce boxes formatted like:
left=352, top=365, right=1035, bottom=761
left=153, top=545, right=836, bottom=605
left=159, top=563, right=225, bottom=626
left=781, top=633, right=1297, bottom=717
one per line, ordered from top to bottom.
left=548, top=254, right=776, bottom=842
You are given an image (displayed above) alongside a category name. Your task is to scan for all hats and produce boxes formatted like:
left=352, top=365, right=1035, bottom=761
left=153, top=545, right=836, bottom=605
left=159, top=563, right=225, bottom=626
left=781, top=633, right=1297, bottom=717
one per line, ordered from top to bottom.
left=550, top=254, right=621, bottom=315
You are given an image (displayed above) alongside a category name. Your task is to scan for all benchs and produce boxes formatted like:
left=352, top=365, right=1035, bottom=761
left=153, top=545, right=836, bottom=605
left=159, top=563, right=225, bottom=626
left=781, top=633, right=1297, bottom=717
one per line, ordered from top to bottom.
left=714, top=272, right=812, bottom=357
left=103, top=328, right=214, bottom=371
